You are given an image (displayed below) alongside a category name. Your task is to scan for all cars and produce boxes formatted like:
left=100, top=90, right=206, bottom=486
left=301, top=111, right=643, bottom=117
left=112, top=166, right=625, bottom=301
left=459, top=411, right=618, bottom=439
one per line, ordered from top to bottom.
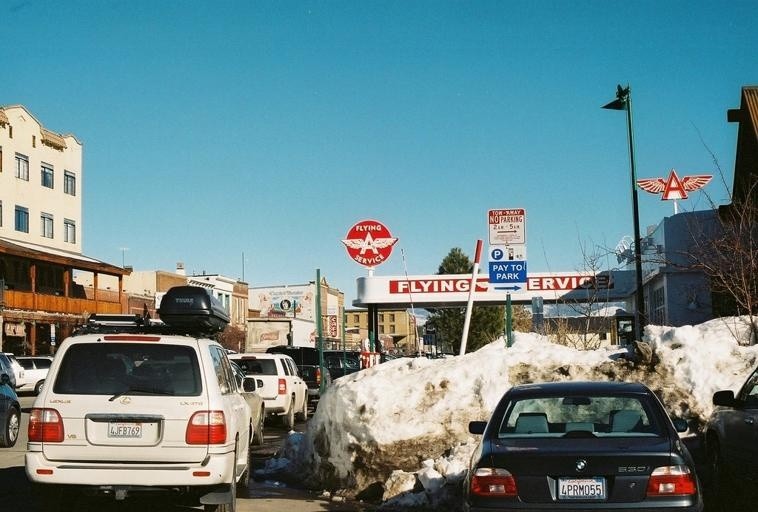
left=25, top=285, right=253, bottom=512
left=13, top=356, right=70, bottom=396
left=230, top=361, right=265, bottom=446
left=699, top=365, right=758, bottom=511
left=0, top=375, right=22, bottom=448
left=462, top=380, right=709, bottom=509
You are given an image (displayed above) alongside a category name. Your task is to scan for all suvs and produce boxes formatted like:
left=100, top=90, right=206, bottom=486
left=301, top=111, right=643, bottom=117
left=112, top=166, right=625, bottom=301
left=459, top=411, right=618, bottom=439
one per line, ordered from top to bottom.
left=265, top=345, right=400, bottom=413
left=0, top=352, right=25, bottom=390
left=227, top=353, right=309, bottom=429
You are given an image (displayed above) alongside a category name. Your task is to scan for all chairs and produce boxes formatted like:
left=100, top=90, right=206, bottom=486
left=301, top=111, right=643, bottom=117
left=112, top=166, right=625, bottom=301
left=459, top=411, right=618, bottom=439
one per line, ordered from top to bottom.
left=513, top=409, right=646, bottom=434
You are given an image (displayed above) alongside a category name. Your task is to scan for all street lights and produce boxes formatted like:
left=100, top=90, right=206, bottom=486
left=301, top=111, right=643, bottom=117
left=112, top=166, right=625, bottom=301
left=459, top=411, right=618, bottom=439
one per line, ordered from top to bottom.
left=600, top=81, right=648, bottom=345
